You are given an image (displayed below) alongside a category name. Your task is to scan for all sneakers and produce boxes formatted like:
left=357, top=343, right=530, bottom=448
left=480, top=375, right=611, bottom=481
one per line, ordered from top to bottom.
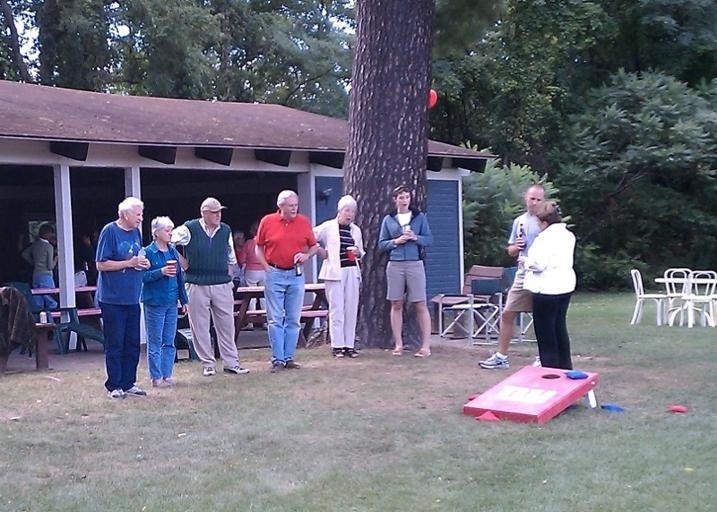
left=392, top=347, right=402, bottom=356
left=332, top=348, right=360, bottom=359
left=414, top=348, right=431, bottom=357
left=478, top=354, right=510, bottom=369
left=267, top=361, right=302, bottom=373
left=222, top=366, right=249, bottom=376
left=202, top=368, right=215, bottom=376
left=107, top=377, right=174, bottom=399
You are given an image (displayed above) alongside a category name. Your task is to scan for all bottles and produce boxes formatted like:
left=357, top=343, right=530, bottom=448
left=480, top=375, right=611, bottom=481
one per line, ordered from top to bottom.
left=516, top=222, right=528, bottom=249
left=134, top=246, right=147, bottom=272
left=295, top=259, right=302, bottom=277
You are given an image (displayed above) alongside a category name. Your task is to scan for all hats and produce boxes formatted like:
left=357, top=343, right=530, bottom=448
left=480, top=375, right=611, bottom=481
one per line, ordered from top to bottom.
left=201, top=198, right=226, bottom=212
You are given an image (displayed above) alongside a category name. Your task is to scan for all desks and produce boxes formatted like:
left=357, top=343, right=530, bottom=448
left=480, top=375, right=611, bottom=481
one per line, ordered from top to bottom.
left=655, top=273, right=717, bottom=327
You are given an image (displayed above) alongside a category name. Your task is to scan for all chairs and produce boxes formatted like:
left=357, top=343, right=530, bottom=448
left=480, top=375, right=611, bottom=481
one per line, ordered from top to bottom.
left=431, top=264, right=531, bottom=345
left=665, top=266, right=694, bottom=323
left=631, top=269, right=667, bottom=324
left=686, top=270, right=716, bottom=328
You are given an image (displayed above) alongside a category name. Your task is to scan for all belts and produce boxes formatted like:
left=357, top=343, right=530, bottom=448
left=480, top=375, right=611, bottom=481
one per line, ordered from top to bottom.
left=268, top=262, right=295, bottom=271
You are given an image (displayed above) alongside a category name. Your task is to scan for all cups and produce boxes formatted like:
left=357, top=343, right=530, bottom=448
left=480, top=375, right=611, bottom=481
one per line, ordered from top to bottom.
left=402, top=224, right=411, bottom=236
left=346, top=246, right=357, bottom=261
left=166, top=260, right=177, bottom=276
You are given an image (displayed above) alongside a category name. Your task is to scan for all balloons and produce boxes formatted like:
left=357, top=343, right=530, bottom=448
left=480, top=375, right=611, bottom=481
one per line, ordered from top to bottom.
left=428, top=89, right=439, bottom=108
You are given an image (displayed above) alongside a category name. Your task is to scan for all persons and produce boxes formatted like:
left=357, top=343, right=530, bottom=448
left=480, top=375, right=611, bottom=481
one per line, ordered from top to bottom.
left=376, top=185, right=432, bottom=357
left=477, top=185, right=544, bottom=368
left=254, top=189, right=319, bottom=374
left=310, top=195, right=366, bottom=357
left=95, top=197, right=151, bottom=400
left=169, top=198, right=250, bottom=376
left=516, top=202, right=577, bottom=370
left=137, top=215, right=189, bottom=388
left=0, top=218, right=265, bottom=331
